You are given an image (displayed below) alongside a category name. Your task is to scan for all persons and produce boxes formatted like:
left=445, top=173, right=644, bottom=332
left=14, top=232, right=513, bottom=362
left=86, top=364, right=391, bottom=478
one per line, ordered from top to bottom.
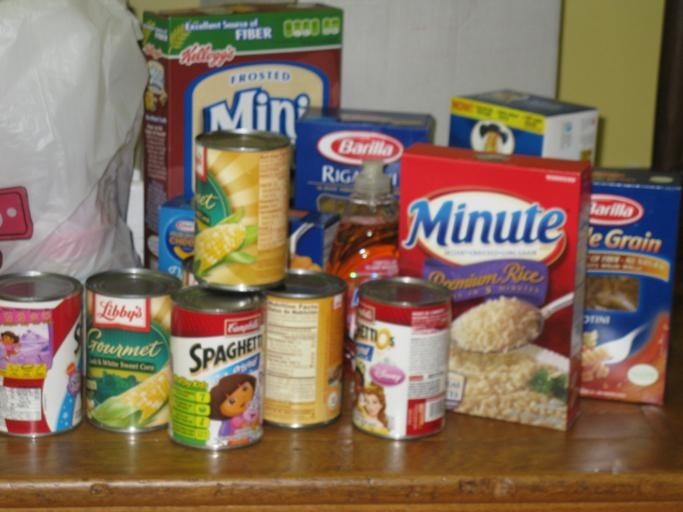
left=477, top=121, right=508, bottom=155
left=353, top=382, right=387, bottom=429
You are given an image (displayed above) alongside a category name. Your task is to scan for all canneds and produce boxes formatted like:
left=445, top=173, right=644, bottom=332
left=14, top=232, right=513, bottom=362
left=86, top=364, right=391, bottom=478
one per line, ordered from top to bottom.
left=193, top=130, right=293, bottom=292
left=84, top=268, right=182, bottom=434
left=260, top=269, right=347, bottom=430
left=0, top=270, right=88, bottom=438
left=354, top=274, right=453, bottom=441
left=170, top=288, right=264, bottom=450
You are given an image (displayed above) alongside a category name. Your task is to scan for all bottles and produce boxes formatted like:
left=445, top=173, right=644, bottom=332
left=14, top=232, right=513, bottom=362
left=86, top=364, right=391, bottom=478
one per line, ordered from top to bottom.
left=323, top=154, right=400, bottom=382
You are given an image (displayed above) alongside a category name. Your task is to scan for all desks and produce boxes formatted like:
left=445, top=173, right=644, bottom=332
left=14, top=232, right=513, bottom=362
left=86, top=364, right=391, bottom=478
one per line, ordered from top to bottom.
left=1, top=312, right=681, bottom=511
